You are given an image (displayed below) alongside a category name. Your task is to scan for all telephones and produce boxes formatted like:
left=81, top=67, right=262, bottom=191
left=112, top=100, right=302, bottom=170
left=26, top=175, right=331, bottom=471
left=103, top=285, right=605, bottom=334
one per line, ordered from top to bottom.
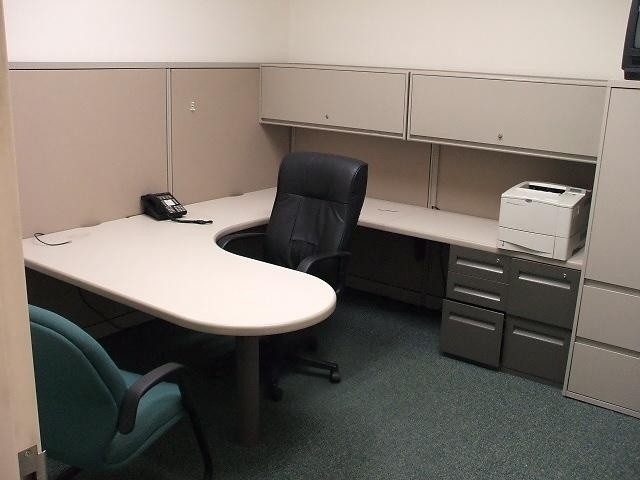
left=140, top=192, right=187, bottom=220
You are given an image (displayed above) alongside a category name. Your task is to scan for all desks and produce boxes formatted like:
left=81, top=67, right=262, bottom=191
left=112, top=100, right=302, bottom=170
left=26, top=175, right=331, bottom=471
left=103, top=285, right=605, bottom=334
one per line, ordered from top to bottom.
left=16, top=184, right=584, bottom=448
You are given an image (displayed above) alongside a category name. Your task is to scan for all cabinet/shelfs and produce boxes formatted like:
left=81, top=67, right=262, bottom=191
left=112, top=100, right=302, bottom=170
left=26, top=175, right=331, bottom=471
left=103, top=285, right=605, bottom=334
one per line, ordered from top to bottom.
left=259, top=65, right=407, bottom=141
left=409, top=68, right=606, bottom=156
left=560, top=78, right=640, bottom=418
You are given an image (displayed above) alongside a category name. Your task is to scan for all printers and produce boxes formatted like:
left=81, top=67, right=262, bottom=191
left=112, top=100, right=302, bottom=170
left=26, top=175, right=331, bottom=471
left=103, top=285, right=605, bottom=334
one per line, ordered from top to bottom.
left=496, top=180, right=592, bottom=261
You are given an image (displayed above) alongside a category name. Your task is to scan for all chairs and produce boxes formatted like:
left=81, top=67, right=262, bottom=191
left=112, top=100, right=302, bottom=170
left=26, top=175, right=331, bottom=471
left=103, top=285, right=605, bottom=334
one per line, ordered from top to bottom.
left=212, top=149, right=370, bottom=401
left=29, top=304, right=215, bottom=480
